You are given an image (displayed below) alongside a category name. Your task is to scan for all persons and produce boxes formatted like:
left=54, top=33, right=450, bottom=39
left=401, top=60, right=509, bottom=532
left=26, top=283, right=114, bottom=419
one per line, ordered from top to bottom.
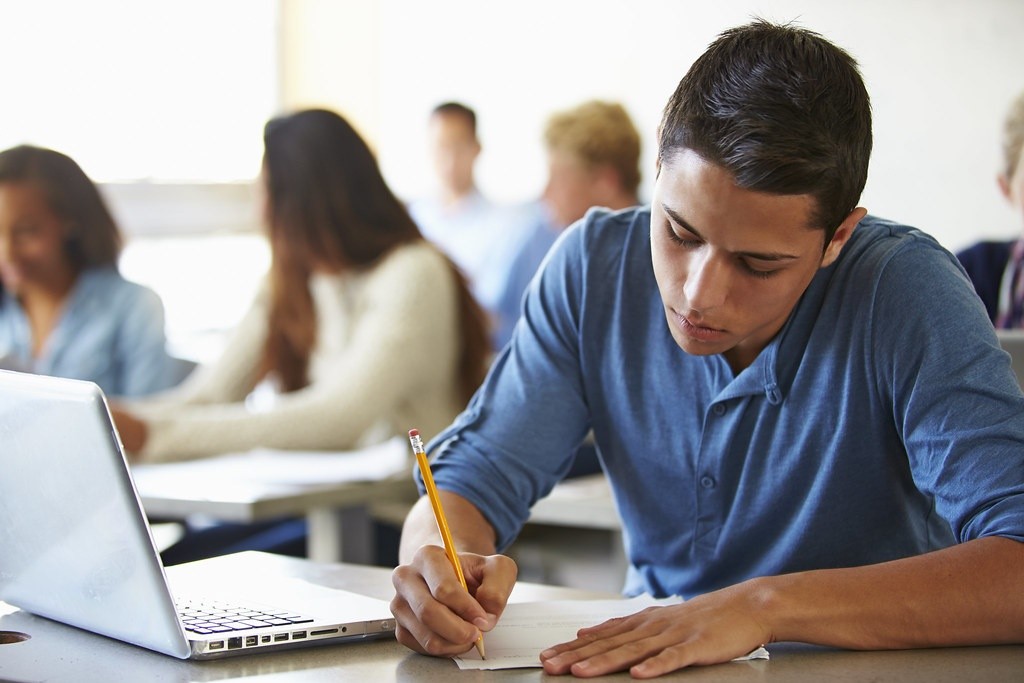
left=390, top=20, right=1024, bottom=683
left=954, top=83, right=1024, bottom=331
left=492, top=98, right=650, bottom=488
left=404, top=99, right=504, bottom=314
left=97, top=109, right=494, bottom=573
left=0, top=144, right=167, bottom=395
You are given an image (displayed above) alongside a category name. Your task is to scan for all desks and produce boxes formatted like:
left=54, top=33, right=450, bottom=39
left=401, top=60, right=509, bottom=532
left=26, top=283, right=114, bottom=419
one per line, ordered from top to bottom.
left=132, top=453, right=417, bottom=565
left=0, top=550, right=1024, bottom=683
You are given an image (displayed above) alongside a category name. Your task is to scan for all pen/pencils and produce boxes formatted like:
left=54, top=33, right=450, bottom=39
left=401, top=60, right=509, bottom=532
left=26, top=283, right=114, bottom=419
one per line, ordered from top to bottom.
left=409, top=428, right=486, bottom=660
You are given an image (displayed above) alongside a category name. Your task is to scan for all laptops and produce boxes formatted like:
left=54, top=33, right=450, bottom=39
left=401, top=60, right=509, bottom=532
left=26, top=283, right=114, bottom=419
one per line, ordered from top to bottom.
left=0, top=370, right=402, bottom=660
